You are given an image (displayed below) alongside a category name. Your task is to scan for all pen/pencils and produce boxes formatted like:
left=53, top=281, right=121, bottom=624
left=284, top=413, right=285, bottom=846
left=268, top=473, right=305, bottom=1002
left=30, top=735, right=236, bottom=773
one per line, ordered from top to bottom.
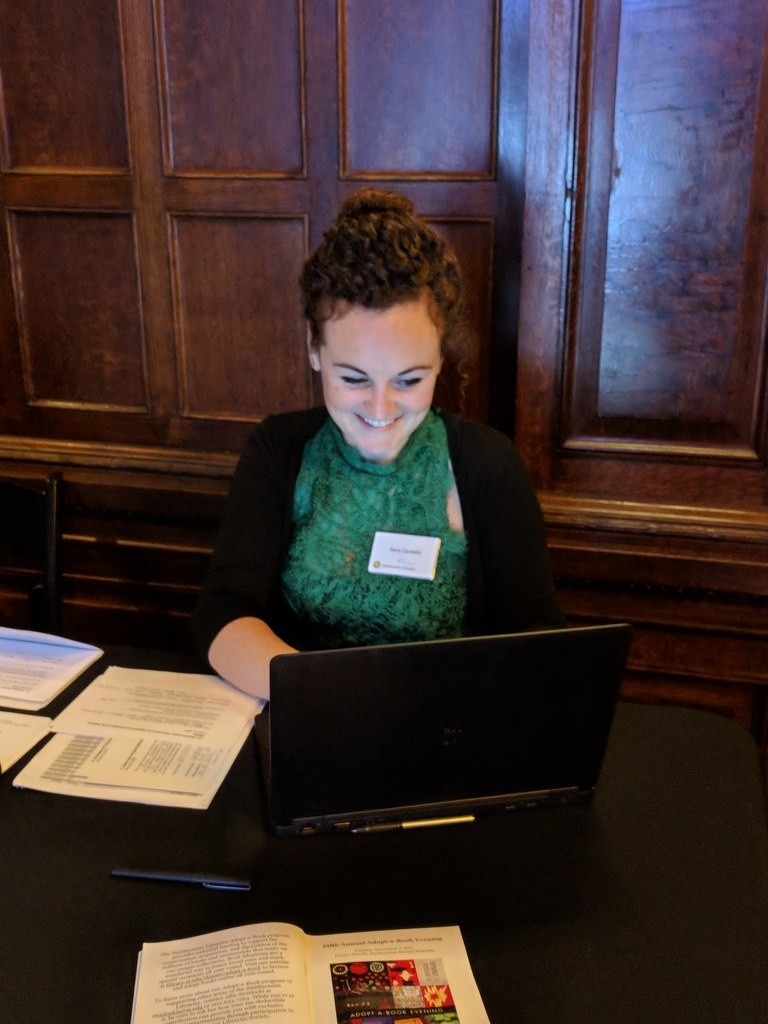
left=110, top=868, right=254, bottom=891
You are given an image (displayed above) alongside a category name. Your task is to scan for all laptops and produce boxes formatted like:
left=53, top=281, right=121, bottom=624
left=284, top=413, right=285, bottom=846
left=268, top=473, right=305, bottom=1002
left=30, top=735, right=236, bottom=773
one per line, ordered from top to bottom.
left=269, top=622, right=635, bottom=836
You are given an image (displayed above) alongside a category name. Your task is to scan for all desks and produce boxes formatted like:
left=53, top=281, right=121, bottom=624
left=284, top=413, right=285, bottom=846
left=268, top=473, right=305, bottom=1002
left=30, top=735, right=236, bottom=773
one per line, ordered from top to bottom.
left=0, top=644, right=768, bottom=1023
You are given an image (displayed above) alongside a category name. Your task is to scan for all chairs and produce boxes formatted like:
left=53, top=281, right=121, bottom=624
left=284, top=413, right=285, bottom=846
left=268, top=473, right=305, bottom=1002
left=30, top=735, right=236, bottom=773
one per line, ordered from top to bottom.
left=1, top=473, right=62, bottom=635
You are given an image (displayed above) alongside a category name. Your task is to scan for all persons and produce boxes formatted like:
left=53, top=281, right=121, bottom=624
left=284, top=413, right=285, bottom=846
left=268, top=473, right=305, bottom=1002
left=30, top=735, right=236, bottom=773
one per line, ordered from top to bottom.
left=190, top=189, right=560, bottom=702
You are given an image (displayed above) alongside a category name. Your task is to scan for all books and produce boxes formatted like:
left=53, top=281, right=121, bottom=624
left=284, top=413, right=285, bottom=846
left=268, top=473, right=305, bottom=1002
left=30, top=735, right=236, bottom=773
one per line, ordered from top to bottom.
left=130, top=923, right=490, bottom=1024
